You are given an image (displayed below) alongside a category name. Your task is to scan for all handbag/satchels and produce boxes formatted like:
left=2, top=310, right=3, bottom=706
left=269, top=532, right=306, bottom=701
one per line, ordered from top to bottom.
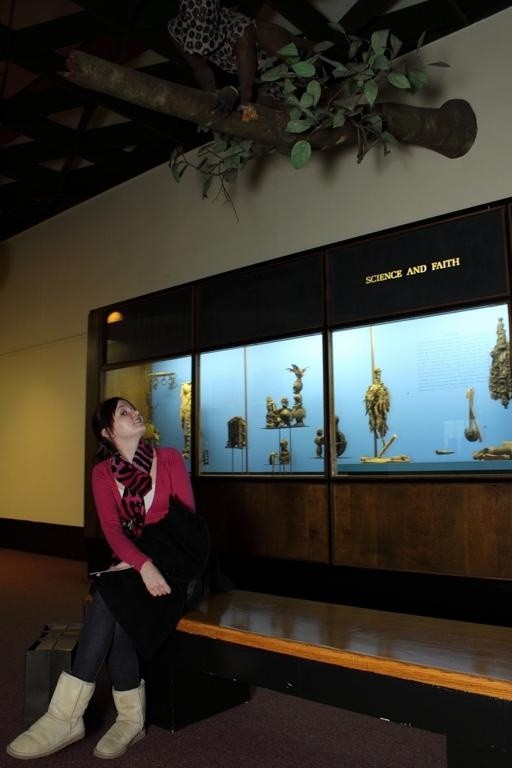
left=23, top=623, right=85, bottom=713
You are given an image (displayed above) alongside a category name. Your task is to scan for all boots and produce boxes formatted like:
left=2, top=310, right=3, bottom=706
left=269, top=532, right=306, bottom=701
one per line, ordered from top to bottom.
left=4, top=671, right=97, bottom=760
left=93, top=679, right=148, bottom=760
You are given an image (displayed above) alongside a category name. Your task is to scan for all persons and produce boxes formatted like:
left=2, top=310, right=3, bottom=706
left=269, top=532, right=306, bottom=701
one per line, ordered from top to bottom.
left=5, top=394, right=202, bottom=763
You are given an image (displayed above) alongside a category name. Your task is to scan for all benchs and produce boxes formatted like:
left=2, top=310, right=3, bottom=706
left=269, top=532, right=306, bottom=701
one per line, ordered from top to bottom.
left=175, top=588, right=512, bottom=768
